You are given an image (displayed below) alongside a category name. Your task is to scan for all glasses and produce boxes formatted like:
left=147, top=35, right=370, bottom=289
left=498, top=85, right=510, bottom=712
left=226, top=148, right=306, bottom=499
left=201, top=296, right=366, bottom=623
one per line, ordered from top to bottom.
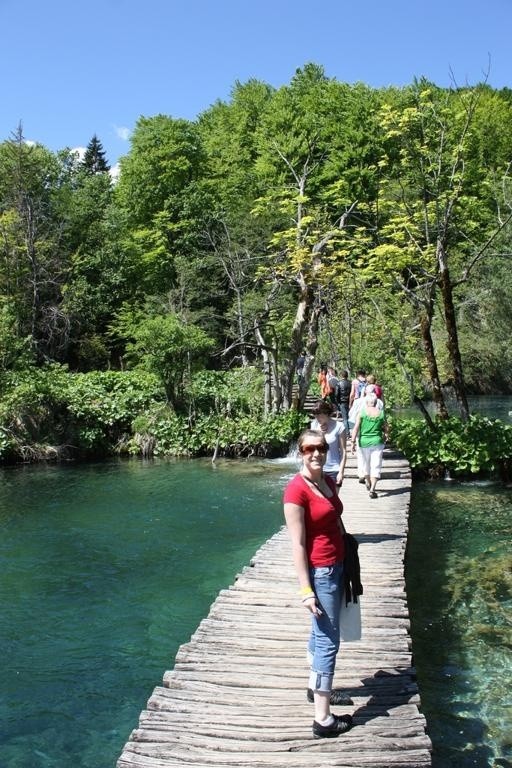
left=299, top=444, right=329, bottom=455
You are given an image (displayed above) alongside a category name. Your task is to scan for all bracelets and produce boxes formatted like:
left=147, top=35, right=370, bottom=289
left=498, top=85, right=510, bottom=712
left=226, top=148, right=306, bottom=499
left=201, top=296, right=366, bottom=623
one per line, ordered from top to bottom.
left=298, top=586, right=316, bottom=603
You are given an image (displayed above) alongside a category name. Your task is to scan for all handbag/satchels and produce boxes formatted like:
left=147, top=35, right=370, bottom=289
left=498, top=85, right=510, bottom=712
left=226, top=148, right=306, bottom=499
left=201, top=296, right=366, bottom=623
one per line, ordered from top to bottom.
left=345, top=533, right=358, bottom=549
left=339, top=582, right=362, bottom=643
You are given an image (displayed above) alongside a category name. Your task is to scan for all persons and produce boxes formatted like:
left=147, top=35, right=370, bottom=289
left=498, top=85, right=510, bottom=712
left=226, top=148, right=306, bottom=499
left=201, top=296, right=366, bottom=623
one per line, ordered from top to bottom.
left=311, top=365, right=389, bottom=497
left=283, top=429, right=363, bottom=737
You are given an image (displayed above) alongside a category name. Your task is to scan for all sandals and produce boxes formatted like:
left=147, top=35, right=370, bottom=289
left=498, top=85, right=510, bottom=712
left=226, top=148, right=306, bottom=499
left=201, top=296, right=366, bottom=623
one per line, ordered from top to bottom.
left=359, top=477, right=377, bottom=498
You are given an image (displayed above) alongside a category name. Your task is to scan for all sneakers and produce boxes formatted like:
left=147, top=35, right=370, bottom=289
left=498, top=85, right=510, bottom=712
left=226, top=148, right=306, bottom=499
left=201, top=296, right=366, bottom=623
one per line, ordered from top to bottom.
left=307, top=688, right=353, bottom=705
left=313, top=714, right=353, bottom=738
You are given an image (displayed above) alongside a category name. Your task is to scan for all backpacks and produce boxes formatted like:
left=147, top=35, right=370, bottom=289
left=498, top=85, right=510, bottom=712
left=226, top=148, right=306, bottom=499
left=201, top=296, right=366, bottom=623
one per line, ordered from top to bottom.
left=356, top=378, right=367, bottom=398
